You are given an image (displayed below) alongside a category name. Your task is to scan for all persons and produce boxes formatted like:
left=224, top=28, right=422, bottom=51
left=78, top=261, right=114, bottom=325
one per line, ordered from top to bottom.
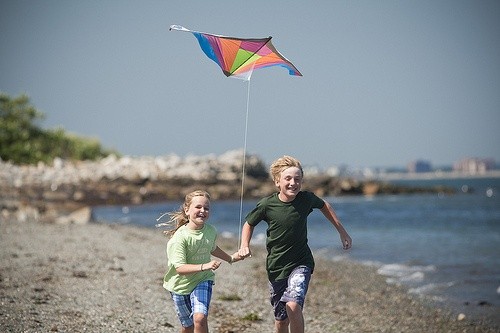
left=238, top=155, right=352, bottom=333
left=163, top=190, right=244, bottom=333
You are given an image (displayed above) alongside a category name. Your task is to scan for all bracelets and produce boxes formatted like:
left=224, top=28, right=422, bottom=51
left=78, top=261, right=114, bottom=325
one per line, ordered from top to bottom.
left=201, top=264, right=204, bottom=271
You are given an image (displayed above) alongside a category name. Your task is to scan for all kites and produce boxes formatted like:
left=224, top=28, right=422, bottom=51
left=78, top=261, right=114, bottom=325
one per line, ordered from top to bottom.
left=169, top=25, right=303, bottom=77
